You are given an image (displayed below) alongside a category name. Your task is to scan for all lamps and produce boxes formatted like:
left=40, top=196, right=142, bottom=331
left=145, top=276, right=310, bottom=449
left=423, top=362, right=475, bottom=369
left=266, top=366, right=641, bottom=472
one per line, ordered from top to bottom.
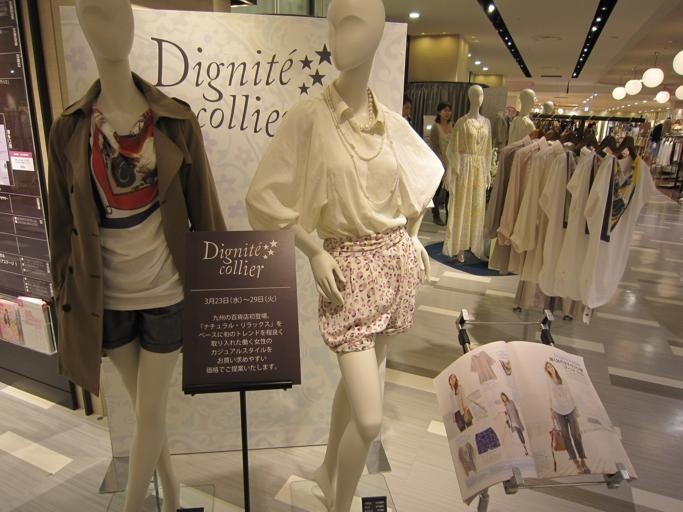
left=612, top=50, right=683, bottom=104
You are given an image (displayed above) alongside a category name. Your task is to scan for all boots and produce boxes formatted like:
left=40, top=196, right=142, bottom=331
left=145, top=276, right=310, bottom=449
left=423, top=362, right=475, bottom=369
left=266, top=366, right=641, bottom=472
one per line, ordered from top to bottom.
left=432, top=207, right=445, bottom=225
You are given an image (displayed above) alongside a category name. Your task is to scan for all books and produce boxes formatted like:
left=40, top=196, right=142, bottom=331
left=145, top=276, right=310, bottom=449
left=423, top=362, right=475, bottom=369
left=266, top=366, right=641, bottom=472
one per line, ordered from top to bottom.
left=430, top=341, right=642, bottom=503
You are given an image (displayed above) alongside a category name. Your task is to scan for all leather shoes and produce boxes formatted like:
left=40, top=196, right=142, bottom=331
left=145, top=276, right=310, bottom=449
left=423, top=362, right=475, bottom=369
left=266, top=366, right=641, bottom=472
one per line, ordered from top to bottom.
left=577, top=467, right=591, bottom=474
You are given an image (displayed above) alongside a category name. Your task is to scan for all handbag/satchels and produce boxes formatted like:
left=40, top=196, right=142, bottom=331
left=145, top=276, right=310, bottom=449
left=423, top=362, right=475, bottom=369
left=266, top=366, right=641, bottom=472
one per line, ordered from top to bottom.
left=463, top=408, right=473, bottom=424
left=506, top=420, right=510, bottom=428
left=550, top=430, right=566, bottom=451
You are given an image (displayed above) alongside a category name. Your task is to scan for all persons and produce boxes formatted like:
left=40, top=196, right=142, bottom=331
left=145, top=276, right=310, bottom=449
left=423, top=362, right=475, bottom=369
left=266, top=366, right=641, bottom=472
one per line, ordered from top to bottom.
left=39, top=0, right=227, bottom=510
left=240, top=0, right=448, bottom=511
left=441, top=82, right=495, bottom=266
left=505, top=87, right=539, bottom=145
left=401, top=94, right=414, bottom=130
left=543, top=100, right=554, bottom=115
left=430, top=101, right=458, bottom=226
left=545, top=360, right=591, bottom=475
left=448, top=372, right=473, bottom=432
left=499, top=391, right=530, bottom=457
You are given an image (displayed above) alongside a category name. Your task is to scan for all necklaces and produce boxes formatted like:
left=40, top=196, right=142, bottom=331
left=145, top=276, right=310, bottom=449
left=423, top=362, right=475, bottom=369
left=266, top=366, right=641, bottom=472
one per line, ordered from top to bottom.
left=326, top=84, right=375, bottom=131
left=320, top=87, right=388, bottom=161
left=322, top=94, right=400, bottom=205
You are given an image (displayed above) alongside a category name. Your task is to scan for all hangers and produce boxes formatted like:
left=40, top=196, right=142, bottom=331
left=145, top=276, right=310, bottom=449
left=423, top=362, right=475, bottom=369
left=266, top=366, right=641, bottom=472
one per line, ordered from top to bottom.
left=529, top=113, right=637, bottom=159
